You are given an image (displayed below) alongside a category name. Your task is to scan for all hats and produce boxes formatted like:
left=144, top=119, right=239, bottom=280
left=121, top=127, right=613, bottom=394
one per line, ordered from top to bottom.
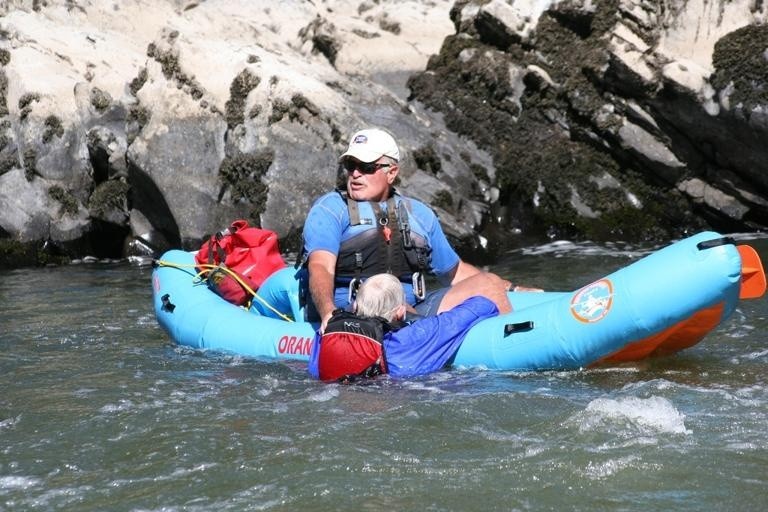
left=339, top=129, right=400, bottom=164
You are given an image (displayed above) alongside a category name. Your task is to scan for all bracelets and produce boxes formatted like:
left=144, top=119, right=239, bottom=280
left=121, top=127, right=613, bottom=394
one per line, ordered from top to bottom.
left=509, top=282, right=519, bottom=292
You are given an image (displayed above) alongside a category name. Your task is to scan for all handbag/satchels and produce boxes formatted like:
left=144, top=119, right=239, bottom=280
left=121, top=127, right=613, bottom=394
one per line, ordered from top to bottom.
left=195, top=220, right=288, bottom=304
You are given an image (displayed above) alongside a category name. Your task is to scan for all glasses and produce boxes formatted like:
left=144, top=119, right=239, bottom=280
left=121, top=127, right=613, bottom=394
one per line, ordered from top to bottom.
left=342, top=158, right=396, bottom=175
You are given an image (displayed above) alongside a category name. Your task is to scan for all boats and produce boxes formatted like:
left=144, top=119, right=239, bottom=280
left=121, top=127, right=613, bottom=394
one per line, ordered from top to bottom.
left=152, top=230, right=768, bottom=370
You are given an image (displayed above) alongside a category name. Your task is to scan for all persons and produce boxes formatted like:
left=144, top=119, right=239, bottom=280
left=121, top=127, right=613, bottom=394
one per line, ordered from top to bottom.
left=301, top=129, right=545, bottom=334
left=307, top=272, right=499, bottom=377
